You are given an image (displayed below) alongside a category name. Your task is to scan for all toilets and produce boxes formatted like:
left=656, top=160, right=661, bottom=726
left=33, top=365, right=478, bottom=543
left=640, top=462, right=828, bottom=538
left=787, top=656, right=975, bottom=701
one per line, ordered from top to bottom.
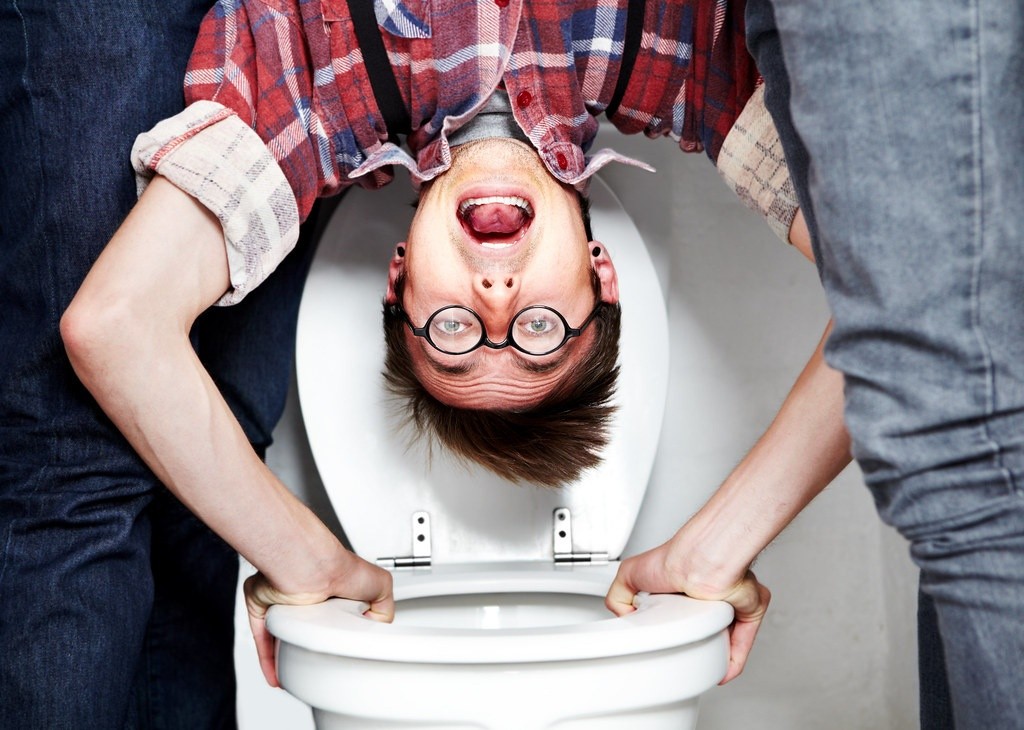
left=263, top=140, right=736, bottom=730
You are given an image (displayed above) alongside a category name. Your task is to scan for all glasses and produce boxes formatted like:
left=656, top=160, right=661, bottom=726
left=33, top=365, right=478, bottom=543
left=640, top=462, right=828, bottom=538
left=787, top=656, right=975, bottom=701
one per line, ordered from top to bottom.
left=398, top=305, right=598, bottom=357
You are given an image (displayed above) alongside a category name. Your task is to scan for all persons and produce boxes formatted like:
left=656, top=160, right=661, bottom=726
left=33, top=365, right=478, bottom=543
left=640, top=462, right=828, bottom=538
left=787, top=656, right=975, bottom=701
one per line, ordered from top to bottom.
left=0, top=0, right=1024, bottom=730
